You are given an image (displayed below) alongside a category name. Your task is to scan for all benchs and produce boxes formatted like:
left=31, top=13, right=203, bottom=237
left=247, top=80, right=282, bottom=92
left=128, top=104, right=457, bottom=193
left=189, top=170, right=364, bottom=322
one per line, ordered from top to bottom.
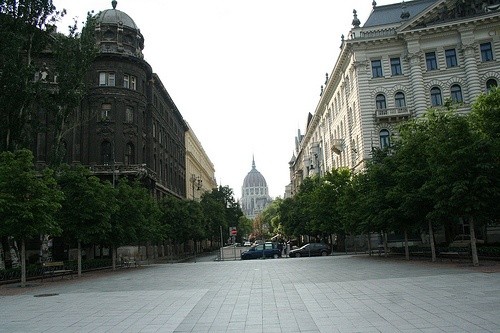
left=40, top=261, right=73, bottom=283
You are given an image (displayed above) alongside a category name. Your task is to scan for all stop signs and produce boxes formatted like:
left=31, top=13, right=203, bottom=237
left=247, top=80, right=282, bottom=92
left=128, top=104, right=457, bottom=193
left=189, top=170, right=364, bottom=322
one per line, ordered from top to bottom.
left=232, top=229, right=237, bottom=235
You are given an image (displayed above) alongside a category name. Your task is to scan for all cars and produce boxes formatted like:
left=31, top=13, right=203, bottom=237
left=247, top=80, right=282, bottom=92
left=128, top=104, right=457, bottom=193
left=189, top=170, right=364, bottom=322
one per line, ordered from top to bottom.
left=449, top=233, right=485, bottom=248
left=240, top=241, right=281, bottom=260
left=244, top=241, right=252, bottom=247
left=289, top=242, right=333, bottom=258
